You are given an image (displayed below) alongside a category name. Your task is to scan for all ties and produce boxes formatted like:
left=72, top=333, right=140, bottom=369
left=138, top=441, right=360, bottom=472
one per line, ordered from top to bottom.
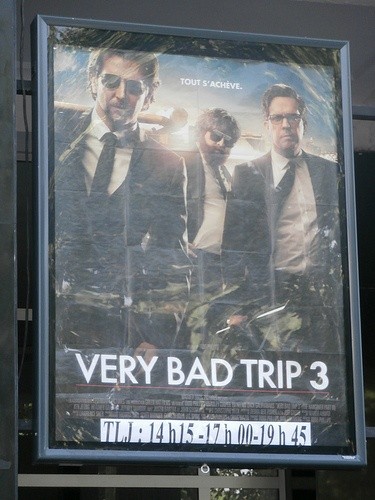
left=88, top=135, right=116, bottom=198
left=269, top=158, right=296, bottom=224
left=211, top=161, right=228, bottom=201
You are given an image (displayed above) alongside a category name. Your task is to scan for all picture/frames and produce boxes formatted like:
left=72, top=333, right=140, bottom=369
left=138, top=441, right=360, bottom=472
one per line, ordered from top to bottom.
left=29, top=13, right=368, bottom=467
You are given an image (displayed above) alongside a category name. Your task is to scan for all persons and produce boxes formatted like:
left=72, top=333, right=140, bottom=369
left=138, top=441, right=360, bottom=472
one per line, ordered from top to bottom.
left=52, top=42, right=187, bottom=391
left=220, top=84, right=340, bottom=353
left=174, top=106, right=242, bottom=353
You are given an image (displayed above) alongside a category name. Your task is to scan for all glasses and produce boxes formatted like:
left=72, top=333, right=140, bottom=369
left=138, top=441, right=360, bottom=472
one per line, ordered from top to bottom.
left=100, top=72, right=145, bottom=95
left=268, top=114, right=301, bottom=123
left=210, top=131, right=234, bottom=147
